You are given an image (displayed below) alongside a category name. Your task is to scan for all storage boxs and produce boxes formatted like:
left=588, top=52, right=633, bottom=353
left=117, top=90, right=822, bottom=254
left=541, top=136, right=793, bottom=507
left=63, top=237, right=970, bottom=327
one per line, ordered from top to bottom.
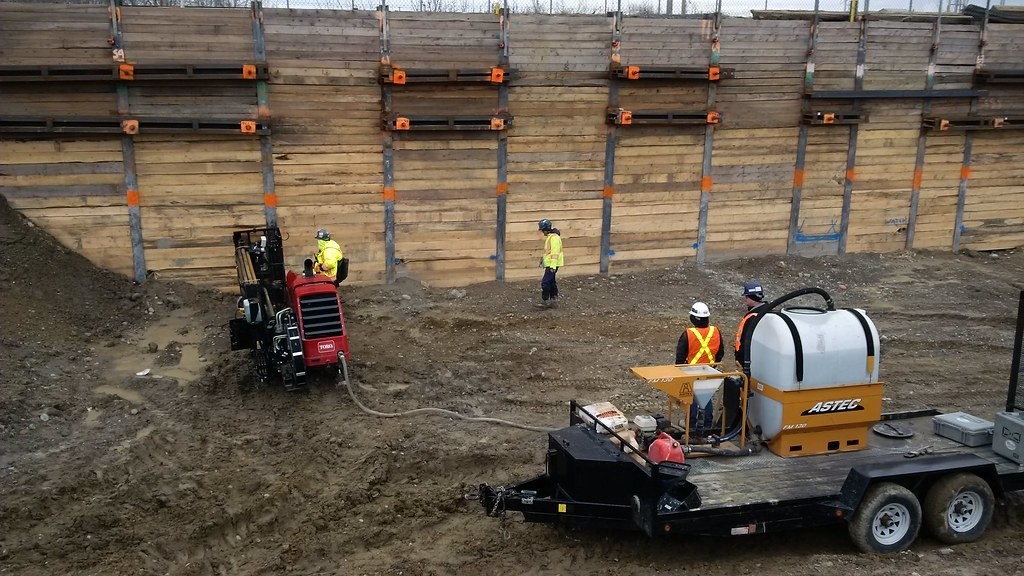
left=931, top=411, right=995, bottom=447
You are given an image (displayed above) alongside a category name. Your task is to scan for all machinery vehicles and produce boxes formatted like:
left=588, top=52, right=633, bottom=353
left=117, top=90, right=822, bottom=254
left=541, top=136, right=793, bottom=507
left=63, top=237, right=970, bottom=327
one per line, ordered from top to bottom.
left=228, top=227, right=350, bottom=394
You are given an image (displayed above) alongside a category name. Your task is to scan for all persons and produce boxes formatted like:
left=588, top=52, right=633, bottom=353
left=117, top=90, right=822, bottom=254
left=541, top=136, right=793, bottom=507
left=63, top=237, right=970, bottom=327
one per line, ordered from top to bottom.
left=535, top=218, right=564, bottom=308
left=314, top=229, right=343, bottom=286
left=734, top=282, right=769, bottom=370
left=675, top=302, right=725, bottom=428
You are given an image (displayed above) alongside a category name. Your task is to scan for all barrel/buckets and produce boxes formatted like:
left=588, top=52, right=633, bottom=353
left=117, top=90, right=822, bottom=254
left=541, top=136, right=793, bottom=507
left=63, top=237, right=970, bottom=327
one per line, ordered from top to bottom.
left=648, top=432, right=684, bottom=464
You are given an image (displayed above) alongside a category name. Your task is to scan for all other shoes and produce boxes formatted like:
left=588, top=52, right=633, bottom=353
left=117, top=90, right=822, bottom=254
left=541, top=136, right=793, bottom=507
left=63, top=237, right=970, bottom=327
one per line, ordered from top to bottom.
left=549, top=300, right=559, bottom=308
left=535, top=302, right=548, bottom=310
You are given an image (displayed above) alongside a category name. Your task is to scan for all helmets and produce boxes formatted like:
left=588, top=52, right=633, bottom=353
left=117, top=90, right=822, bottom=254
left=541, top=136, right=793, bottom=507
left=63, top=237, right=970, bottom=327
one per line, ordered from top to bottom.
left=538, top=220, right=553, bottom=229
left=314, top=230, right=329, bottom=239
left=741, top=284, right=764, bottom=295
left=689, top=302, right=710, bottom=318
